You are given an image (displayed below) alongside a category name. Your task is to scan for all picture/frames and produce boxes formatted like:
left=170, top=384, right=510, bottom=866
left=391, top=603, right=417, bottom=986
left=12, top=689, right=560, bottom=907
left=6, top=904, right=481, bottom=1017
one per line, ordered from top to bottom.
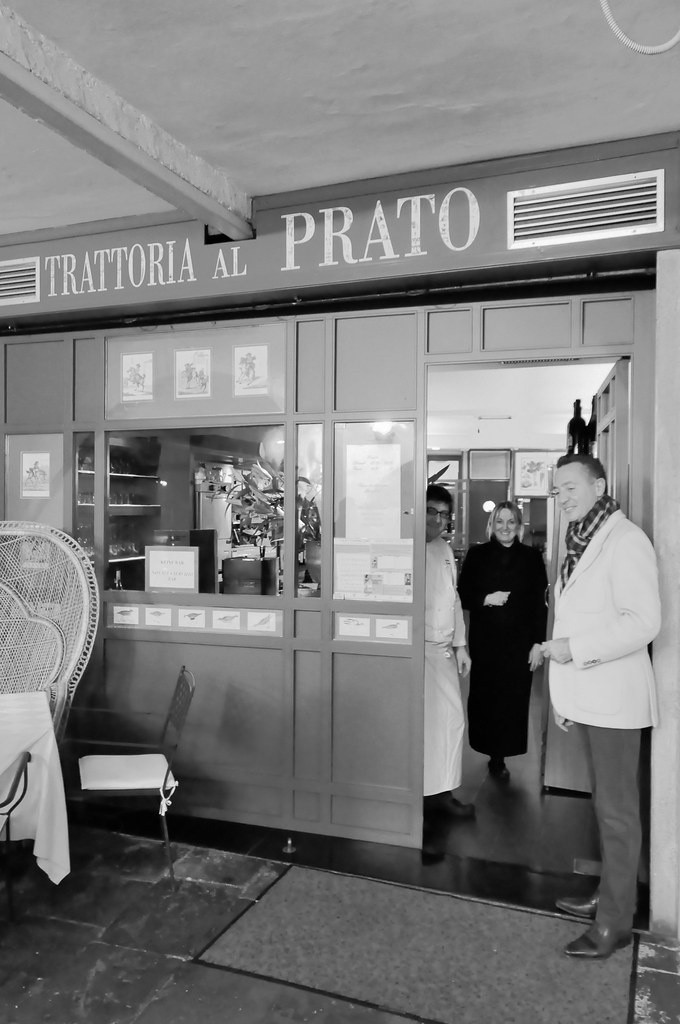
left=232, top=343, right=272, bottom=397
left=173, top=347, right=212, bottom=400
left=119, top=351, right=156, bottom=404
left=510, top=448, right=567, bottom=499
left=18, top=450, right=52, bottom=501
left=143, top=546, right=199, bottom=593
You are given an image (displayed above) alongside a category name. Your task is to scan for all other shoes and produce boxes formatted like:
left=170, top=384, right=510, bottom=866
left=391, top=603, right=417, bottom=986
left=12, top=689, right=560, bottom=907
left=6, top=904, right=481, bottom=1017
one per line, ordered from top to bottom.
left=489, top=762, right=510, bottom=780
left=440, top=798, right=475, bottom=817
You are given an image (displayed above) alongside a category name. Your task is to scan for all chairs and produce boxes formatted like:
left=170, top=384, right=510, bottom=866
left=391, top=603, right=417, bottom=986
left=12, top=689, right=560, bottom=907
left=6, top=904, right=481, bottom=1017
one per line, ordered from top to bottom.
left=0, top=520, right=99, bottom=742
left=77, top=664, right=196, bottom=893
left=0, top=751, right=48, bottom=921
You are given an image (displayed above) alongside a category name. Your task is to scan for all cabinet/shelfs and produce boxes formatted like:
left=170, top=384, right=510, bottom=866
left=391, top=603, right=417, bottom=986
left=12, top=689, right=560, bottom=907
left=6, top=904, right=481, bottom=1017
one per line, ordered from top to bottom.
left=74, top=433, right=161, bottom=591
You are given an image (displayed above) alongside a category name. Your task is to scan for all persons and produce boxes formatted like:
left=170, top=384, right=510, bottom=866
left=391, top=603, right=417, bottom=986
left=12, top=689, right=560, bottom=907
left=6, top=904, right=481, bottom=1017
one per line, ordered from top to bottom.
left=540, top=454, right=663, bottom=960
left=422, top=483, right=475, bottom=821
left=457, top=501, right=548, bottom=781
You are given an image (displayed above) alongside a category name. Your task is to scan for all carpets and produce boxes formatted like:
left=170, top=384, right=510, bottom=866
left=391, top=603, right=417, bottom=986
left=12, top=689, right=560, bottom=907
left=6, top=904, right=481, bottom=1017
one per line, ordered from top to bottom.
left=192, top=865, right=641, bottom=1024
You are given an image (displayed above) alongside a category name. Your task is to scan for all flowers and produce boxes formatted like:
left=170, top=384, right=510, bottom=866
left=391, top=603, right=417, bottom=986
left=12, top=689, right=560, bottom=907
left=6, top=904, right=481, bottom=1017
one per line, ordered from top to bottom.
left=215, top=463, right=321, bottom=543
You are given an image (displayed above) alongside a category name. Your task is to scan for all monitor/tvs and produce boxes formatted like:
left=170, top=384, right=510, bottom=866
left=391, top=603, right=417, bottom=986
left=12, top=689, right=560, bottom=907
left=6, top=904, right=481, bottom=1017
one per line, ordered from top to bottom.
left=154, top=529, right=220, bottom=594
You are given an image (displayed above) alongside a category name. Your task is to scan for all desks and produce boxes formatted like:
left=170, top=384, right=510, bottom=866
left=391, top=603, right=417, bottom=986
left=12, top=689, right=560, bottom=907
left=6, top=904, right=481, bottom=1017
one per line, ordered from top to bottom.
left=0, top=691, right=71, bottom=885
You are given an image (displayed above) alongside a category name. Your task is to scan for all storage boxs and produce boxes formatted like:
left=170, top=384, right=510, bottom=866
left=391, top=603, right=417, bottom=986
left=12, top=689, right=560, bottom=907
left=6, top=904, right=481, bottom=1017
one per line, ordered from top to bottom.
left=189, top=528, right=219, bottom=594
left=222, top=557, right=279, bottom=597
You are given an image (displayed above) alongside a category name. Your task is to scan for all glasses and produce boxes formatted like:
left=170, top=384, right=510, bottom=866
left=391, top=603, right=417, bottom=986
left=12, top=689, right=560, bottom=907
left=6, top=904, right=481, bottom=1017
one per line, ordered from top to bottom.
left=427, top=507, right=450, bottom=519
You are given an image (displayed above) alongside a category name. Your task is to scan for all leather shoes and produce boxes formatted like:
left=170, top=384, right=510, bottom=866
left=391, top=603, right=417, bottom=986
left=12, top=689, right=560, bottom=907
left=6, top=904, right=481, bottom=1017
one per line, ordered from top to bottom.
left=555, top=891, right=601, bottom=918
left=565, top=923, right=632, bottom=959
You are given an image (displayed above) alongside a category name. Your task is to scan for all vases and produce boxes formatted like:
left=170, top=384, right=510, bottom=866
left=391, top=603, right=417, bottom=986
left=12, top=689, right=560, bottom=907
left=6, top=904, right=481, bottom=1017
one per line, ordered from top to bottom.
left=305, top=541, right=321, bottom=583
